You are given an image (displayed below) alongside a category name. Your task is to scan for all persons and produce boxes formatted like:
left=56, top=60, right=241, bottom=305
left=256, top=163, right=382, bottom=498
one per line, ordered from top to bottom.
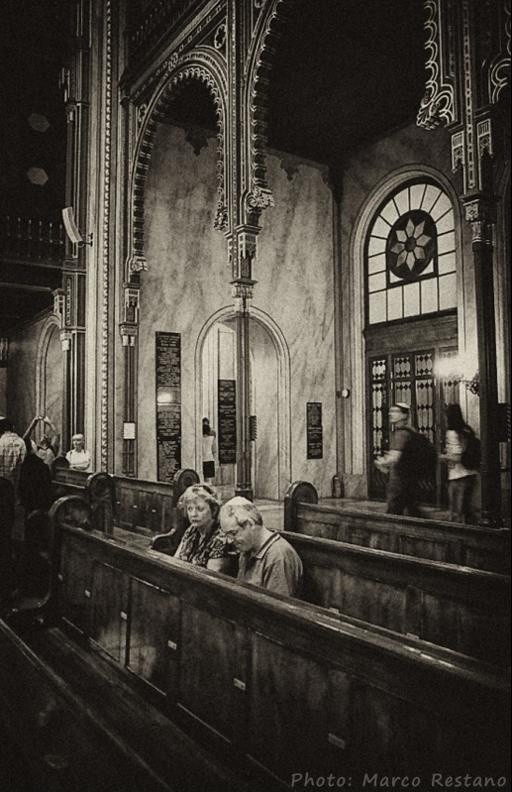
left=373, top=402, right=432, bottom=518
left=437, top=402, right=479, bottom=524
left=0, top=415, right=26, bottom=512
left=21, top=415, right=61, bottom=470
left=201, top=415, right=217, bottom=459
left=200, top=425, right=217, bottom=484
left=65, top=434, right=91, bottom=471
left=171, top=481, right=237, bottom=574
left=220, top=496, right=304, bottom=600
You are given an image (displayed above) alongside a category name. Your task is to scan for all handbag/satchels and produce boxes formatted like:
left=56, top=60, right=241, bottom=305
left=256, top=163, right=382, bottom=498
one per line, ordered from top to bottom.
left=393, top=426, right=438, bottom=485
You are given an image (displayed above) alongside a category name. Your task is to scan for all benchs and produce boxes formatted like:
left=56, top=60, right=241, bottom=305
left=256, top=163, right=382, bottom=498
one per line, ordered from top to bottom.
left=0, top=465, right=512, bottom=791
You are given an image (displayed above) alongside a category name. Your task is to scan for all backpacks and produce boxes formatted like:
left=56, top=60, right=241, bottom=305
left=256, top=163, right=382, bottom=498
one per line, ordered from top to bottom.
left=456, top=428, right=482, bottom=471
